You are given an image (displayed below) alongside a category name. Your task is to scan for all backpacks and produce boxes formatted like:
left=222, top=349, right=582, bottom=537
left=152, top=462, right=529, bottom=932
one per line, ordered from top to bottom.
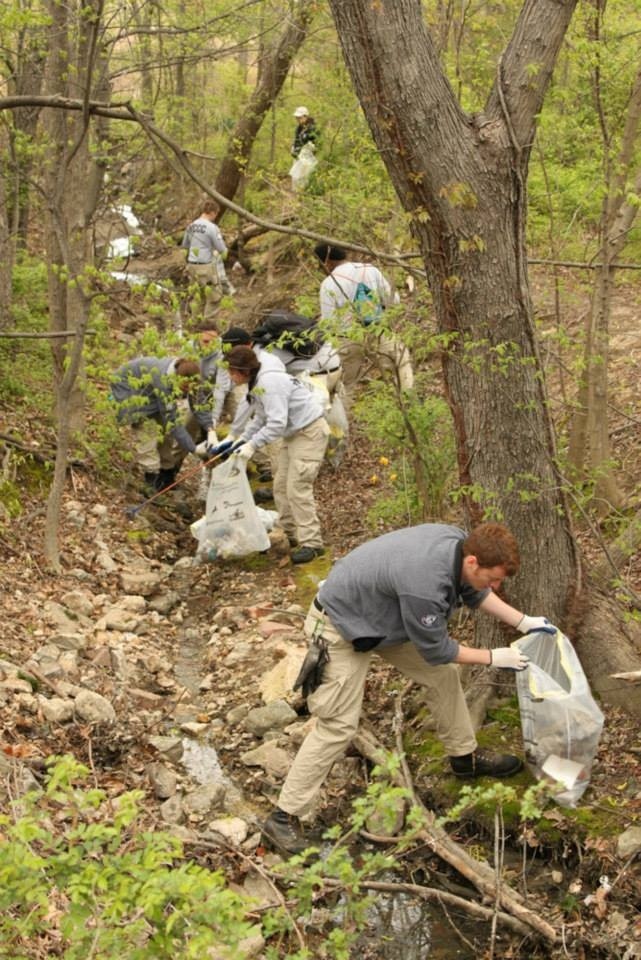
left=331, top=262, right=386, bottom=325
left=251, top=313, right=323, bottom=358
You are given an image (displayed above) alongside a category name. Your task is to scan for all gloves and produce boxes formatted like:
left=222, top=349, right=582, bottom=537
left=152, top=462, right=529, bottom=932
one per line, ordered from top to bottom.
left=205, top=431, right=219, bottom=447
left=211, top=438, right=233, bottom=459
left=194, top=440, right=207, bottom=457
left=233, top=441, right=255, bottom=461
left=515, top=614, right=557, bottom=634
left=487, top=648, right=529, bottom=670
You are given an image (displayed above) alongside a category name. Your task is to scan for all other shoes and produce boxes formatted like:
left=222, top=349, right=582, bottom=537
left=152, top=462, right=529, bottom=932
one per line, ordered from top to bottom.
left=254, top=488, right=274, bottom=501
left=294, top=546, right=323, bottom=562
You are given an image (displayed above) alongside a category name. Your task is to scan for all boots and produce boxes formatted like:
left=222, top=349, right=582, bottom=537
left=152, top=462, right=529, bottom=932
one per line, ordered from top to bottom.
left=146, top=466, right=175, bottom=491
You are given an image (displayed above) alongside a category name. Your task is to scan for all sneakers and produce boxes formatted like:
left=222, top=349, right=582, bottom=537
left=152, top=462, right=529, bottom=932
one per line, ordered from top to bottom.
left=450, top=749, right=522, bottom=776
left=260, top=814, right=319, bottom=863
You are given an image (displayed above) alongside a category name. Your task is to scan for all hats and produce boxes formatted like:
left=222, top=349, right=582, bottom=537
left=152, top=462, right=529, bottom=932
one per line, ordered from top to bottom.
left=221, top=327, right=249, bottom=343
left=222, top=344, right=260, bottom=370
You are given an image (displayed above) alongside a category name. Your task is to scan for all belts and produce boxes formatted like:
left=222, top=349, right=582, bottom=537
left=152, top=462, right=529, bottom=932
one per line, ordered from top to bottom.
left=188, top=261, right=211, bottom=264
left=310, top=367, right=339, bottom=376
left=312, top=596, right=326, bottom=613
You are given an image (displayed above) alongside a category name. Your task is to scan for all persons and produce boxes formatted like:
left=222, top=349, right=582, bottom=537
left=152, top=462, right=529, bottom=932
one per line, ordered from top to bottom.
left=291, top=107, right=320, bottom=158
left=182, top=199, right=225, bottom=329
left=315, top=242, right=413, bottom=405
left=172, top=320, right=274, bottom=482
left=206, top=328, right=348, bottom=501
left=210, top=346, right=331, bottom=564
left=109, top=354, right=218, bottom=489
left=260, top=523, right=558, bottom=865
